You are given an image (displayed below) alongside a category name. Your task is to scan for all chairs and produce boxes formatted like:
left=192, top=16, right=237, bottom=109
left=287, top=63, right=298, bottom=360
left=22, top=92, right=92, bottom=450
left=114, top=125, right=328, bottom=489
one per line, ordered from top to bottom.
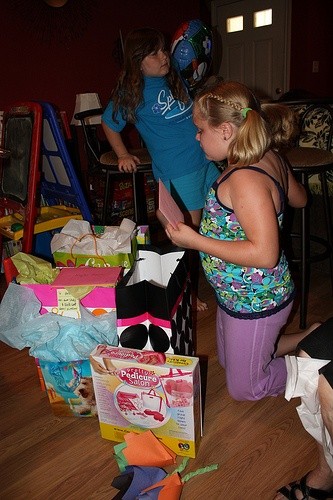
left=277, top=103, right=333, bottom=329
left=74, top=105, right=153, bottom=226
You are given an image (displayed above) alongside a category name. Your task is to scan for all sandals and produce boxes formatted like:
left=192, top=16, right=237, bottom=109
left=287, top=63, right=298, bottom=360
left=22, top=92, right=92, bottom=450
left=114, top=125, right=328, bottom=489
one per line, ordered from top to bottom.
left=272, top=471, right=333, bottom=500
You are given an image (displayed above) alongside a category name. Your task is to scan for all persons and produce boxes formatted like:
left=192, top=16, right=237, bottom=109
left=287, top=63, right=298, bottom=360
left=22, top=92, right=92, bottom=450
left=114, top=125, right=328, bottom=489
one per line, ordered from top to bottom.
left=101, top=26, right=333, bottom=500
left=90, top=356, right=119, bottom=377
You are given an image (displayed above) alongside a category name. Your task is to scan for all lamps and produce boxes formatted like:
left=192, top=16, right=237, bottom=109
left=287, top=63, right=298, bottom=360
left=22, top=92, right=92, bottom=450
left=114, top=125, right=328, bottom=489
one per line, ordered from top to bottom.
left=71, top=93, right=103, bottom=163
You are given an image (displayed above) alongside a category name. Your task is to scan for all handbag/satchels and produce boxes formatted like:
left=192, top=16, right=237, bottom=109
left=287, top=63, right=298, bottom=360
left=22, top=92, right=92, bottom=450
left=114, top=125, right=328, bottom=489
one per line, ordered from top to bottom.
left=4, top=217, right=205, bottom=459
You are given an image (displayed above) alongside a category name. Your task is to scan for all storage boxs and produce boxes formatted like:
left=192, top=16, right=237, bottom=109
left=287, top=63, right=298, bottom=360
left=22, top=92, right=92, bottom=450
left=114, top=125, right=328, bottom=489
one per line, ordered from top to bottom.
left=88, top=344, right=203, bottom=459
